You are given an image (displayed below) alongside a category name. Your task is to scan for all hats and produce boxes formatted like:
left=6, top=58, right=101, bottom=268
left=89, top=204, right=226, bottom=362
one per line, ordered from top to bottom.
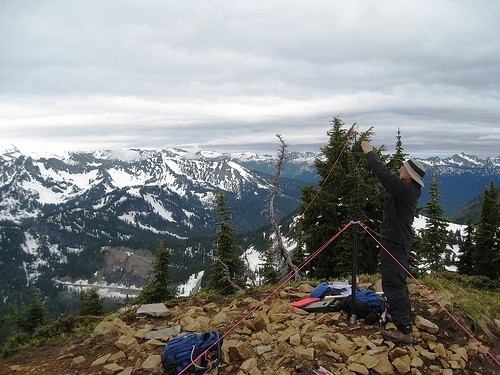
left=403, top=158, right=426, bottom=187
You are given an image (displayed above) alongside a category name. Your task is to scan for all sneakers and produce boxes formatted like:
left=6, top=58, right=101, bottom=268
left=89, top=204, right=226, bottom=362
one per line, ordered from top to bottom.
left=380, top=332, right=412, bottom=344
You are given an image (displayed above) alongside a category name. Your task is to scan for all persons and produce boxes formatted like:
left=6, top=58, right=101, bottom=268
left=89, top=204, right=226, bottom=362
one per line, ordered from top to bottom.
left=359, top=141, right=427, bottom=346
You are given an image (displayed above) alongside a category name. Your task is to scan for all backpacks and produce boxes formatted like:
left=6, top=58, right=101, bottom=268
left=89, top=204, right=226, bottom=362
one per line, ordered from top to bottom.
left=343, top=286, right=386, bottom=322
left=159, top=329, right=224, bottom=371
